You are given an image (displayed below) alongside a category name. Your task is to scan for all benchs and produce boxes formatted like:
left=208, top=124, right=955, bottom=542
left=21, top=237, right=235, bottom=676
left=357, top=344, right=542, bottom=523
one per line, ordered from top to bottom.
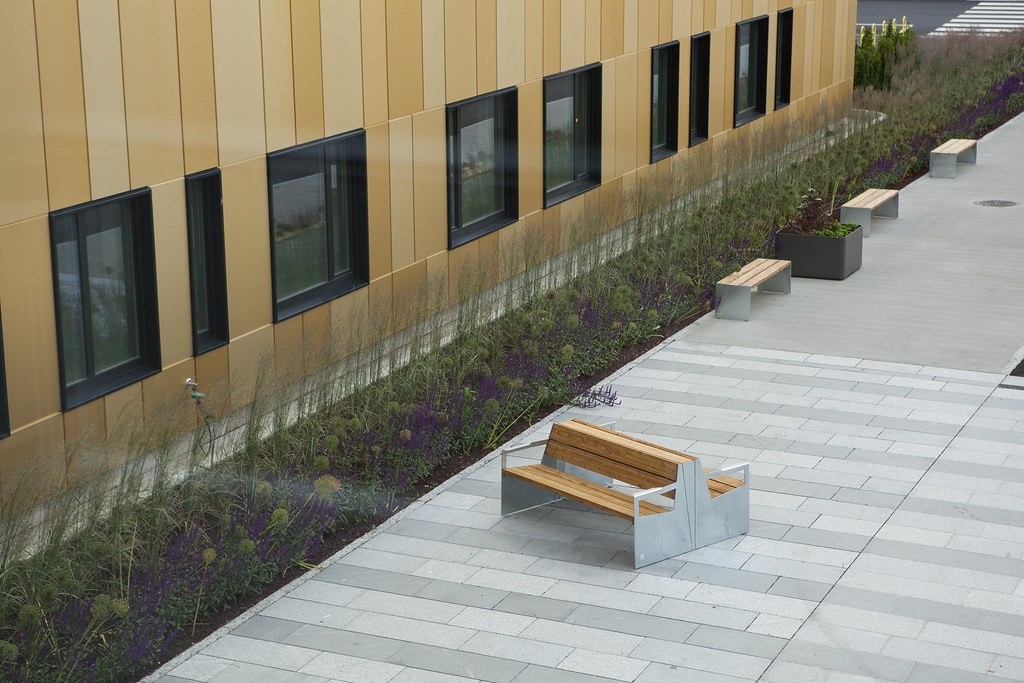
left=929, top=138, right=977, bottom=178
left=839, top=187, right=900, bottom=238
left=715, top=257, right=792, bottom=321
left=564, top=417, right=750, bottom=551
left=499, top=419, right=695, bottom=570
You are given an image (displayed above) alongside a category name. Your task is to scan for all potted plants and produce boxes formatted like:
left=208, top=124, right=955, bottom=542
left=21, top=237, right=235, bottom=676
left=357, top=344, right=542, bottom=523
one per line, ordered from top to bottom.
left=774, top=188, right=863, bottom=280
